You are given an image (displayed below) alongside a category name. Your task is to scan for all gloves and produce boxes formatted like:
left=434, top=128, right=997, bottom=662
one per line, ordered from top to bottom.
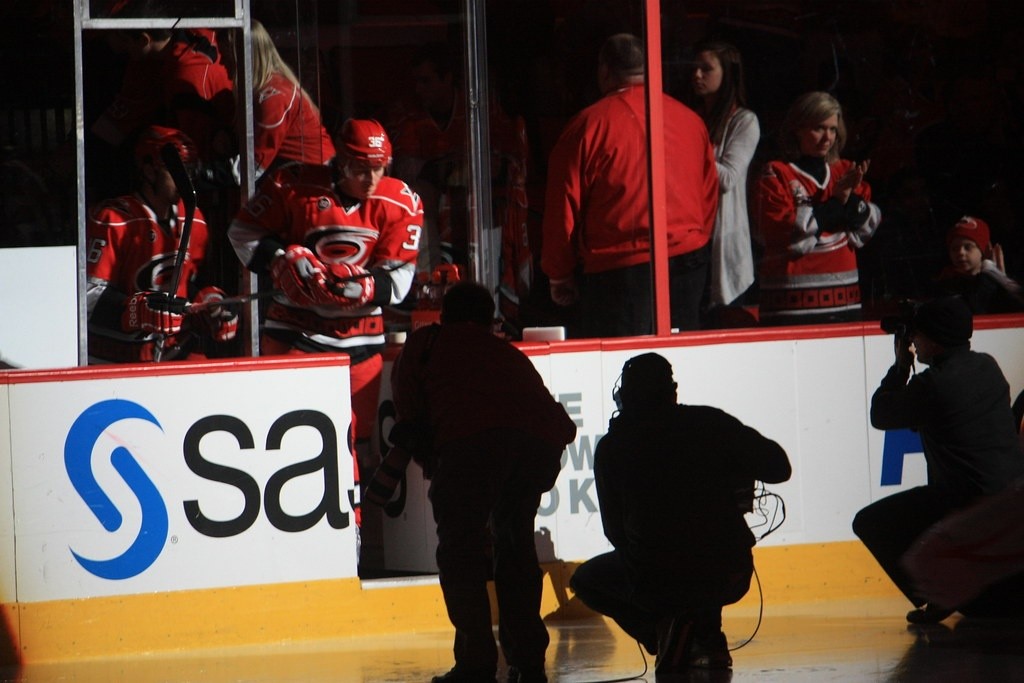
left=272, top=243, right=376, bottom=307
left=191, top=286, right=239, bottom=342
left=120, top=290, right=193, bottom=335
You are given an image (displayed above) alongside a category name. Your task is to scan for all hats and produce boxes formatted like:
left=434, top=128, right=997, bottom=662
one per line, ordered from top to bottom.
left=946, top=215, right=991, bottom=258
left=915, top=294, right=974, bottom=348
left=622, top=353, right=675, bottom=402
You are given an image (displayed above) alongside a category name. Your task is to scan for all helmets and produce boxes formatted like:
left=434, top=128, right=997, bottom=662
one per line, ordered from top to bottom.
left=335, top=116, right=393, bottom=169
left=133, top=126, right=201, bottom=180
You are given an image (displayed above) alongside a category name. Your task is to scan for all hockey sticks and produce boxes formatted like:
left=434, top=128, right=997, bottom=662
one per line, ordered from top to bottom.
left=153, top=142, right=196, bottom=363
left=208, top=225, right=452, bottom=307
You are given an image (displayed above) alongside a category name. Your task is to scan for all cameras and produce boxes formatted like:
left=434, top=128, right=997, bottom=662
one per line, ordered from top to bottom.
left=881, top=299, right=918, bottom=344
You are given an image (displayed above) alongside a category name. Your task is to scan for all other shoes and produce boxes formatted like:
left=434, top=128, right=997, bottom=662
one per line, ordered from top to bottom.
left=907, top=601, right=984, bottom=623
left=653, top=617, right=733, bottom=671
left=507, top=663, right=548, bottom=683
left=431, top=664, right=498, bottom=683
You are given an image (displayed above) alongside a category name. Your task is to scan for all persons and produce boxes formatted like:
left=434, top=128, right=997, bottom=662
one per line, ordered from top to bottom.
left=43, top=9, right=531, bottom=365
left=544, top=33, right=719, bottom=335
left=690, top=46, right=761, bottom=328
left=749, top=92, right=880, bottom=323
left=935, top=214, right=1023, bottom=313
left=377, top=284, right=577, bottom=683
left=850, top=297, right=1019, bottom=623
left=570, top=353, right=791, bottom=669
